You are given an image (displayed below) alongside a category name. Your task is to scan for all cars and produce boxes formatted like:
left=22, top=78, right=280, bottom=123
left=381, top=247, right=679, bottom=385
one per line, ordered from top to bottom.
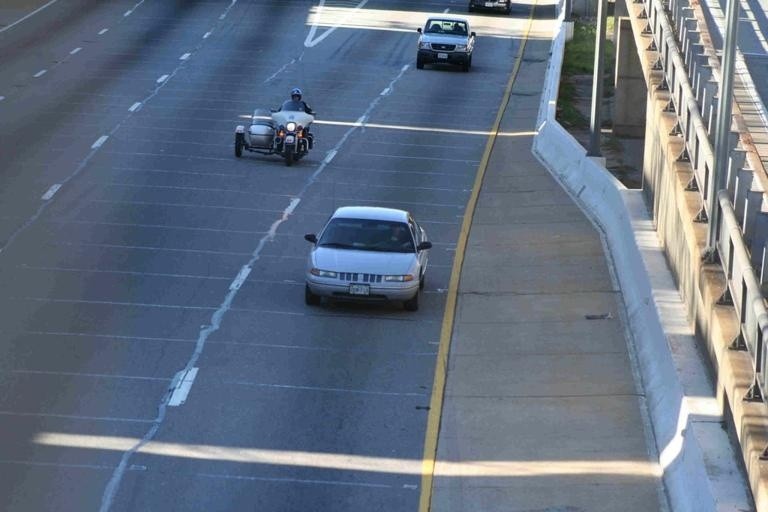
left=469, top=0, right=512, bottom=15
left=304, top=205, right=432, bottom=312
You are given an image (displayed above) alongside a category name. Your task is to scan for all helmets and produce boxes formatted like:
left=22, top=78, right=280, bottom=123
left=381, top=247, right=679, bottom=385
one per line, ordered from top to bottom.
left=291, top=88, right=303, bottom=101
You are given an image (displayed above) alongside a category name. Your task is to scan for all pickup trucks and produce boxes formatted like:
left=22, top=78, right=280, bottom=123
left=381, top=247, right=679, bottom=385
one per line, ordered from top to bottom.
left=416, top=16, right=476, bottom=72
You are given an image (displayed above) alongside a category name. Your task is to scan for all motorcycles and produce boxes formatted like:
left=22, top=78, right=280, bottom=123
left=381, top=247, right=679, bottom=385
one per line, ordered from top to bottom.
left=234, top=104, right=317, bottom=167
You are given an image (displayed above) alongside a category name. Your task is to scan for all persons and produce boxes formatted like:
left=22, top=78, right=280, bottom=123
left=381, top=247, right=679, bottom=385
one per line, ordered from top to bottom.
left=454, top=23, right=464, bottom=35
left=269, top=87, right=313, bottom=150
left=385, top=224, right=412, bottom=249
